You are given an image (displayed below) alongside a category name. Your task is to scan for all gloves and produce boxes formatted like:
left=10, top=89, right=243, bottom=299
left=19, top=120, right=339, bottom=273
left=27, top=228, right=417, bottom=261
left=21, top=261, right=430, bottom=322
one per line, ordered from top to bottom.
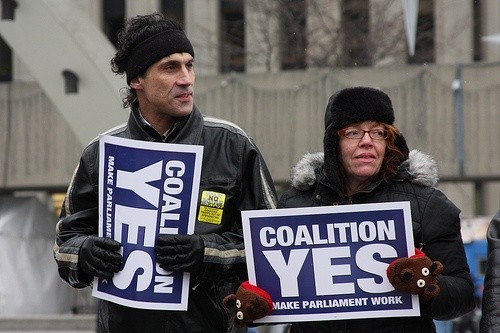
left=155, top=235, right=203, bottom=274
left=80, top=236, right=123, bottom=278
left=387, top=249, right=443, bottom=297
left=222, top=281, right=273, bottom=324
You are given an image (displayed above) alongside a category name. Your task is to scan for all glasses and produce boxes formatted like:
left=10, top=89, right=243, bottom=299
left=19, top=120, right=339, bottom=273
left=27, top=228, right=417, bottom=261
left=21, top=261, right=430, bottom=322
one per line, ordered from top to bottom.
left=338, top=128, right=389, bottom=140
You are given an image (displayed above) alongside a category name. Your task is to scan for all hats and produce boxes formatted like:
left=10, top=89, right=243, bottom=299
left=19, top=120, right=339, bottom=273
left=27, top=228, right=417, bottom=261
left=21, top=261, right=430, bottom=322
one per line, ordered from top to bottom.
left=323, top=86, right=409, bottom=181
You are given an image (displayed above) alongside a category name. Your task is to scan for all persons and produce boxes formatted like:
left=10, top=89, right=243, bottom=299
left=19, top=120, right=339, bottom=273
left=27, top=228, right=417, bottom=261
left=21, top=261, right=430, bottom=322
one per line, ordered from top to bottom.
left=51, top=11, right=280, bottom=333
left=223, top=87, right=476, bottom=333
left=481, top=210, right=500, bottom=333
